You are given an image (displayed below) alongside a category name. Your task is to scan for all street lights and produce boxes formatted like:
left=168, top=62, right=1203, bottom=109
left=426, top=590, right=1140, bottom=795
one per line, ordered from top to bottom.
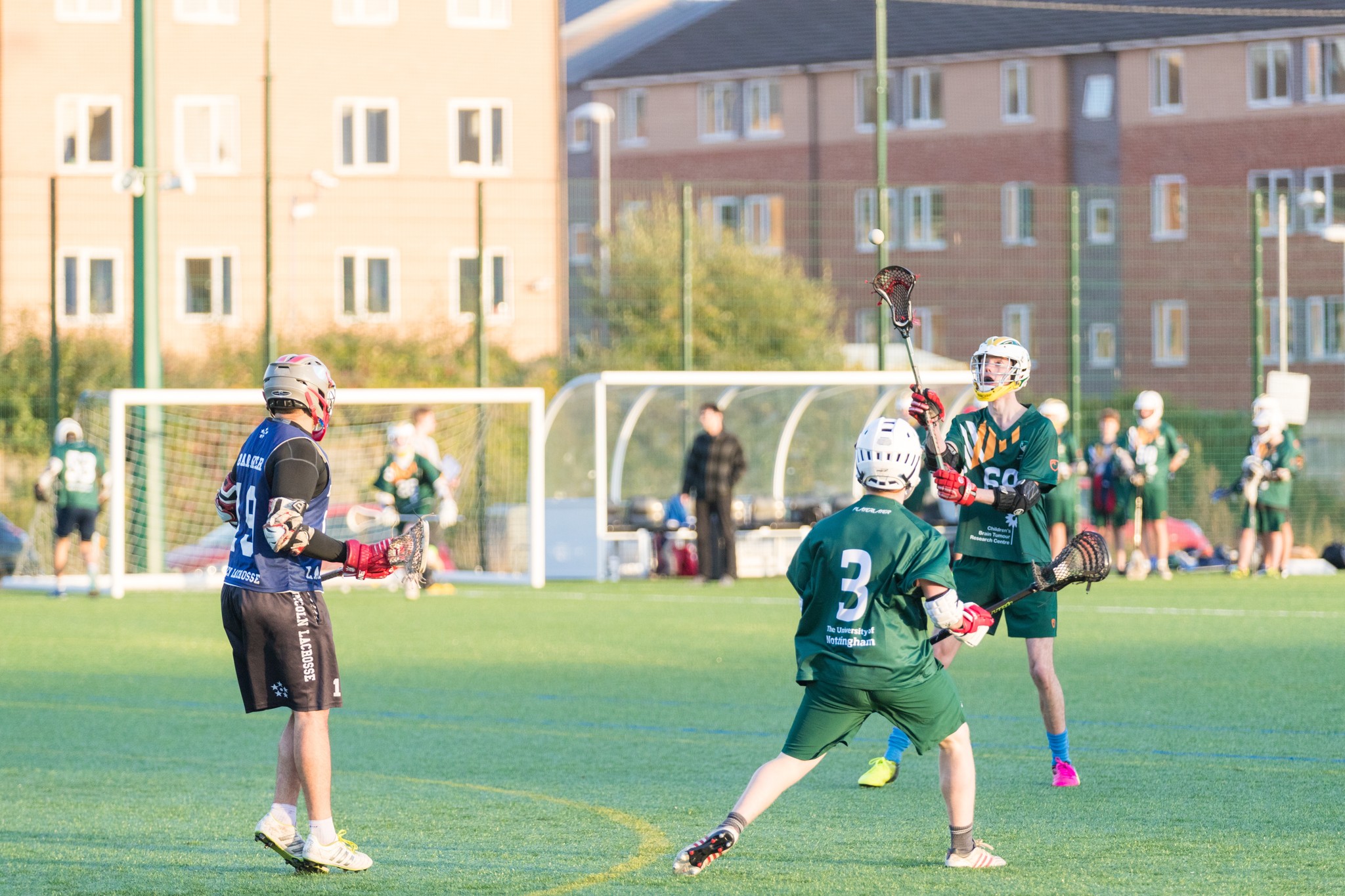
left=572, top=101, right=614, bottom=354
left=1275, top=190, right=1326, bottom=372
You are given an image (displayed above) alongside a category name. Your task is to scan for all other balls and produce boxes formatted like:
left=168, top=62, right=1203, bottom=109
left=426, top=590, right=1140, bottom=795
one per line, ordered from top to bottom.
left=868, top=229, right=885, bottom=245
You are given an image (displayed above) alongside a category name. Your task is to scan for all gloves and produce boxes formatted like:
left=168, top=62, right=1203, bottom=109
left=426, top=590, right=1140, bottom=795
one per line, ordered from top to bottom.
left=437, top=498, right=459, bottom=530
left=382, top=505, right=399, bottom=528
left=343, top=534, right=414, bottom=581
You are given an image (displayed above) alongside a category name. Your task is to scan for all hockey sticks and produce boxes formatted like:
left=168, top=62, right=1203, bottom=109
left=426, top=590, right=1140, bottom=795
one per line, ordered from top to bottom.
left=872, top=265, right=946, bottom=471
left=1125, top=486, right=1151, bottom=581
left=1249, top=502, right=1258, bottom=580
left=345, top=505, right=467, bottom=534
left=321, top=520, right=430, bottom=582
left=930, top=531, right=1112, bottom=645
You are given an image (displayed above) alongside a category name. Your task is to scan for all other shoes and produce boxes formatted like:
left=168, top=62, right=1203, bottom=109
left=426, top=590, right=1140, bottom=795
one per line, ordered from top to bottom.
left=427, top=545, right=444, bottom=575
left=1115, top=560, right=1128, bottom=575
left=1157, top=566, right=1173, bottom=579
left=1228, top=567, right=1250, bottom=578
left=1265, top=568, right=1279, bottom=577
left=689, top=574, right=708, bottom=586
left=714, top=575, right=734, bottom=586
left=403, top=577, right=420, bottom=599
left=89, top=579, right=101, bottom=596
left=52, top=587, right=66, bottom=597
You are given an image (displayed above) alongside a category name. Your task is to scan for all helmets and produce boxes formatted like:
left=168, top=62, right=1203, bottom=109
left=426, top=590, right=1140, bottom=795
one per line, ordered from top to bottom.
left=969, top=335, right=1031, bottom=402
left=1132, top=389, right=1163, bottom=427
left=1248, top=393, right=1286, bottom=444
left=1036, top=398, right=1070, bottom=430
left=855, top=416, right=921, bottom=491
left=52, top=417, right=83, bottom=446
left=385, top=419, right=418, bottom=469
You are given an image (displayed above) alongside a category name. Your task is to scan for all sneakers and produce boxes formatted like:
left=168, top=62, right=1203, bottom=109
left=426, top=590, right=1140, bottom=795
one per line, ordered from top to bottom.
left=857, top=756, right=900, bottom=788
left=1052, top=757, right=1080, bottom=787
left=254, top=811, right=329, bottom=874
left=671, top=825, right=739, bottom=877
left=301, top=830, right=372, bottom=874
left=946, top=838, right=1007, bottom=868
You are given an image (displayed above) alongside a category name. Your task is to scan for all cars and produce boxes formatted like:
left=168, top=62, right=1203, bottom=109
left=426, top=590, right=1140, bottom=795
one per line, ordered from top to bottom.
left=1074, top=476, right=1216, bottom=579
left=161, top=501, right=460, bottom=574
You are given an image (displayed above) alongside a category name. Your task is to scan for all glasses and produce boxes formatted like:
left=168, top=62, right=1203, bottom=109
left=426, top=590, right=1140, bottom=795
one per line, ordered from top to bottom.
left=262, top=353, right=336, bottom=441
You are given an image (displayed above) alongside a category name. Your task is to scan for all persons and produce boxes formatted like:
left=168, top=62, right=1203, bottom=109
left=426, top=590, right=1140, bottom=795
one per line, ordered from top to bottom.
left=858, top=336, right=1082, bottom=790
left=215, top=354, right=397, bottom=873
left=914, top=390, right=1191, bottom=580
left=1228, top=396, right=1308, bottom=581
left=657, top=401, right=749, bottom=586
left=374, top=410, right=462, bottom=600
left=34, top=417, right=108, bottom=596
left=672, top=418, right=1007, bottom=878
